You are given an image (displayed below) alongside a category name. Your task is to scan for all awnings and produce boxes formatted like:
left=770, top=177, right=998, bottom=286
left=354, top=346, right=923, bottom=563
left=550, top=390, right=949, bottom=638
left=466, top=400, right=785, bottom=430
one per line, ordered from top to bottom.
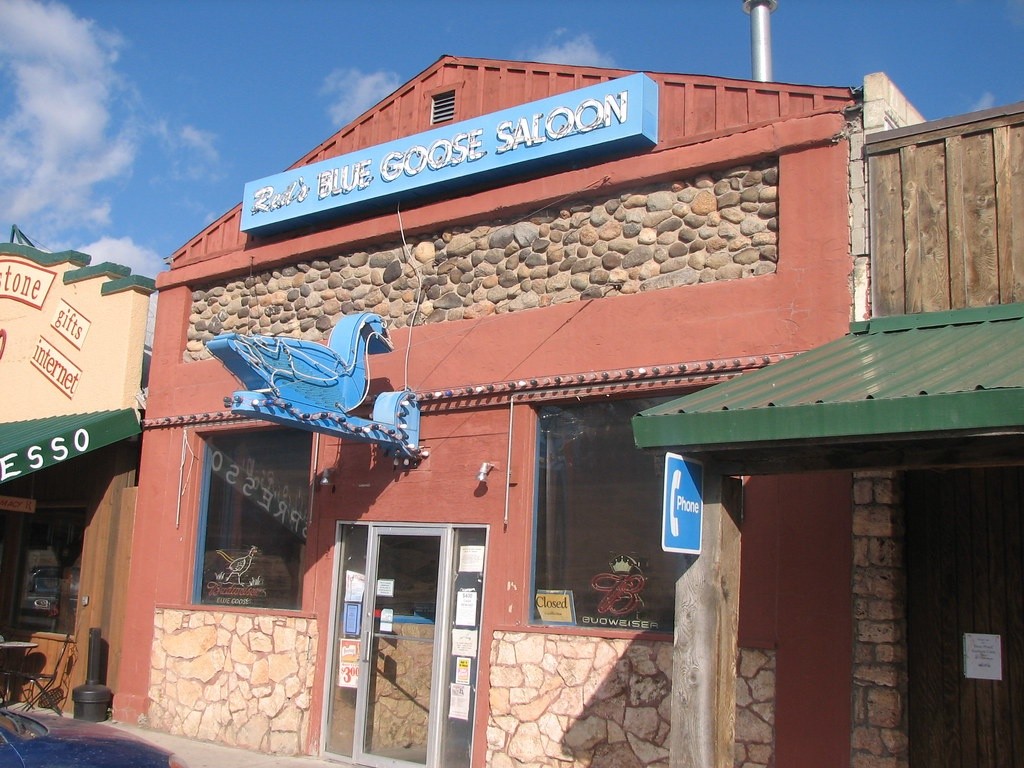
left=631, top=302, right=1024, bottom=449
left=0, top=407, right=143, bottom=485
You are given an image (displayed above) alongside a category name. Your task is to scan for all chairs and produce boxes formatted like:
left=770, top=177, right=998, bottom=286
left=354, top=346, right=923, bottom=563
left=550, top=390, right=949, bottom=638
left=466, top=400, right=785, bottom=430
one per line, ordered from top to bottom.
left=0, top=633, right=71, bottom=717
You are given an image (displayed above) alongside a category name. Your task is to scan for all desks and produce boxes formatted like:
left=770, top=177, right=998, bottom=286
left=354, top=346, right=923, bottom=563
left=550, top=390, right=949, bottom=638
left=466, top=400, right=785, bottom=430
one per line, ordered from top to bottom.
left=0, top=642, right=39, bottom=710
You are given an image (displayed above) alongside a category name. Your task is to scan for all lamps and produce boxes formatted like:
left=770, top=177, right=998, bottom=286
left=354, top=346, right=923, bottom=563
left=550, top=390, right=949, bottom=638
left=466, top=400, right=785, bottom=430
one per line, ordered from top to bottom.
left=475, top=461, right=500, bottom=482
left=321, top=464, right=338, bottom=485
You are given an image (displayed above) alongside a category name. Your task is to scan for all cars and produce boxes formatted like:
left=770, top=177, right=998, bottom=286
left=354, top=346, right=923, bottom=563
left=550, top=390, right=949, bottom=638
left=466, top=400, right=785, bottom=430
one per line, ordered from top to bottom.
left=0, top=706, right=191, bottom=768
